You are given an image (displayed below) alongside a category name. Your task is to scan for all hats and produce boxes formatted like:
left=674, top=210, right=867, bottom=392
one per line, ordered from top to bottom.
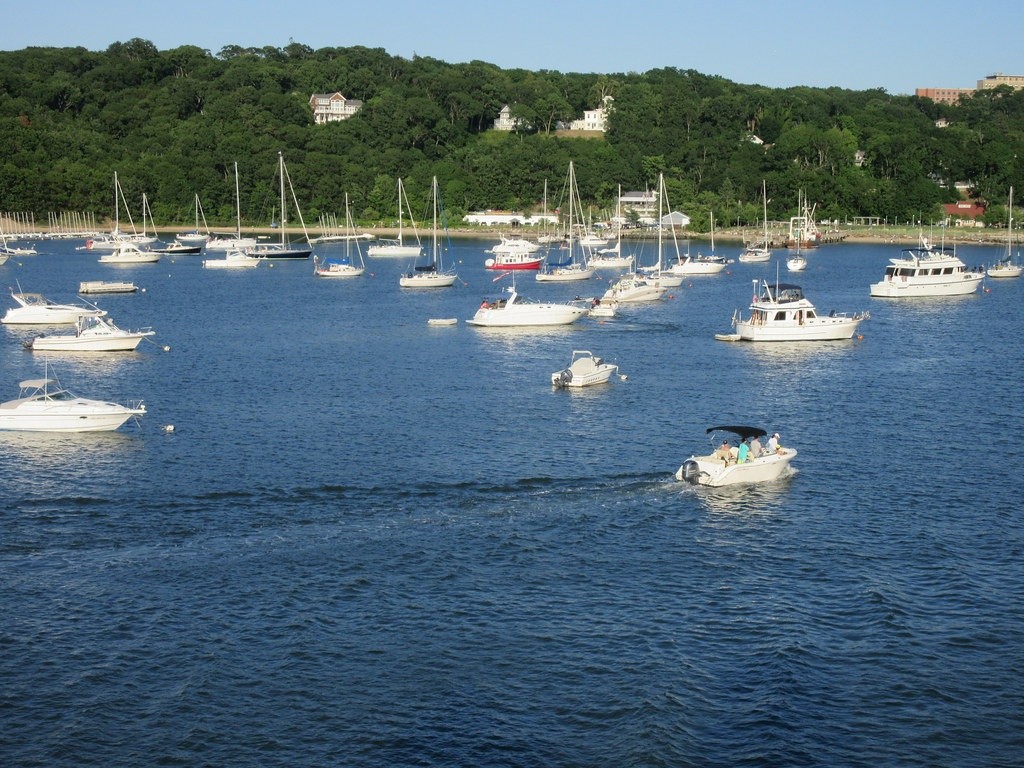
left=775, top=433, right=780, bottom=438
left=723, top=440, right=727, bottom=444
left=775, top=445, right=780, bottom=448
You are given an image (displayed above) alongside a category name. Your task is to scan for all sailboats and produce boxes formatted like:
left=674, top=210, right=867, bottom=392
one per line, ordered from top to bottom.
left=245, top=157, right=312, bottom=260
left=484, top=160, right=808, bottom=287
left=85, top=171, right=211, bottom=242
left=400, top=177, right=459, bottom=286
left=366, top=179, right=422, bottom=257
left=987, top=186, right=1021, bottom=278
left=313, top=192, right=365, bottom=277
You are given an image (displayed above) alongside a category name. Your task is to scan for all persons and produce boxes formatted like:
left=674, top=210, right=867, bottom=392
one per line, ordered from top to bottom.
left=591, top=297, right=601, bottom=307
left=718, top=432, right=786, bottom=465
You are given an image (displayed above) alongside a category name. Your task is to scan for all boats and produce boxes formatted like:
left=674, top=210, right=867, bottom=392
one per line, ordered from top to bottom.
left=206, top=237, right=256, bottom=252
left=88, top=240, right=140, bottom=251
left=96, top=242, right=163, bottom=268
left=146, top=243, right=202, bottom=256
left=0, top=245, right=37, bottom=266
left=205, top=251, right=261, bottom=267
left=870, top=253, right=984, bottom=299
left=603, top=277, right=684, bottom=302
left=79, top=280, right=138, bottom=294
left=734, top=284, right=862, bottom=342
left=0, top=290, right=109, bottom=325
left=590, top=290, right=619, bottom=316
left=465, top=299, right=589, bottom=327
left=33, top=320, right=156, bottom=351
left=551, top=350, right=618, bottom=386
left=0, top=380, right=146, bottom=432
left=672, top=426, right=795, bottom=487
